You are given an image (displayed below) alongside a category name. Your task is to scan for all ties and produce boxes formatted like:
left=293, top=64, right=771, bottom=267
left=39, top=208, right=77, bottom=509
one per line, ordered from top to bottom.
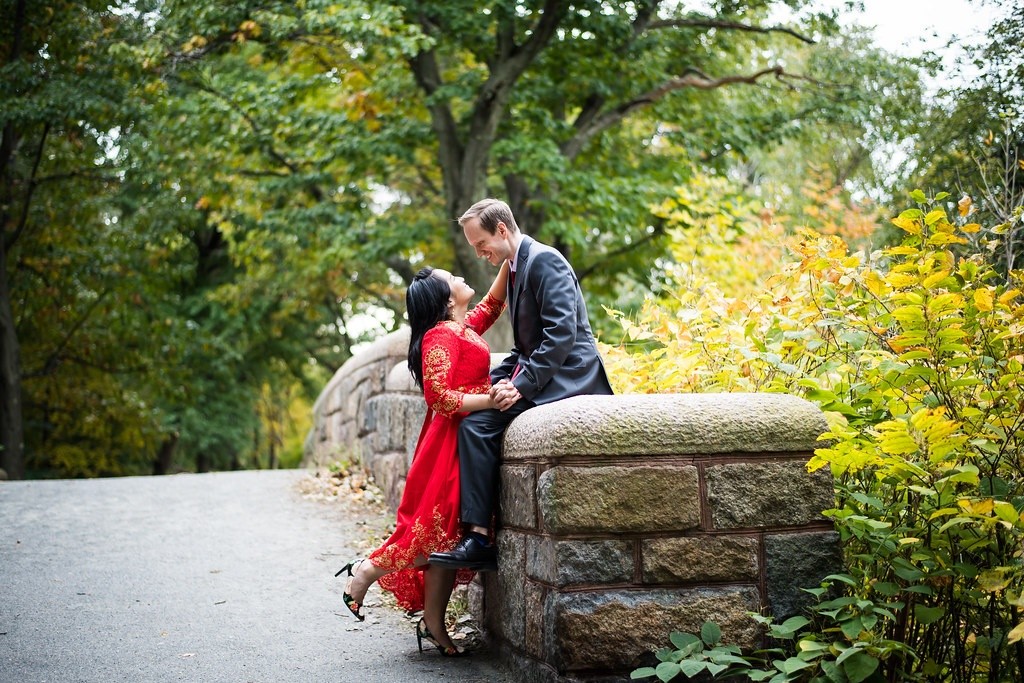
left=510, top=271, right=516, bottom=289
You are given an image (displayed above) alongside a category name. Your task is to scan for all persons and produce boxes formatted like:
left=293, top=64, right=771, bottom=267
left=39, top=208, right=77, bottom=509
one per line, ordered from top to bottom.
left=428, top=198, right=613, bottom=572
left=335, top=259, right=522, bottom=658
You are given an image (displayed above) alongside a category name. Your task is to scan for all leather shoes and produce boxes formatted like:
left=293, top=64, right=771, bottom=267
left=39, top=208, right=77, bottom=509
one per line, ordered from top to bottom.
left=427, top=534, right=500, bottom=571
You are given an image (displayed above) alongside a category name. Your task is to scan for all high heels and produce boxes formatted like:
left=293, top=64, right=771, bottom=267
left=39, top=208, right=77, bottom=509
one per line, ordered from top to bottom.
left=335, top=559, right=365, bottom=622
left=416, top=616, right=472, bottom=658
